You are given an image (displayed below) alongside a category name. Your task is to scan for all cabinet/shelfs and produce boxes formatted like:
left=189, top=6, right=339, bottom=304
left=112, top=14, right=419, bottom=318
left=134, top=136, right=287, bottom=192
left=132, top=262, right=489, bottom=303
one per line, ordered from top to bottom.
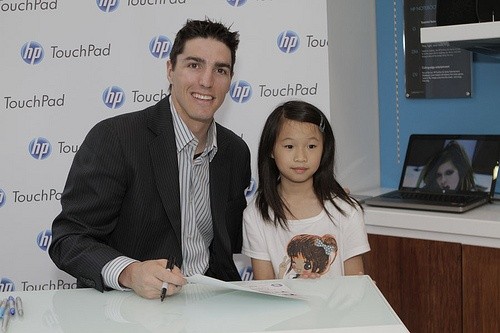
left=362, top=198, right=500, bottom=333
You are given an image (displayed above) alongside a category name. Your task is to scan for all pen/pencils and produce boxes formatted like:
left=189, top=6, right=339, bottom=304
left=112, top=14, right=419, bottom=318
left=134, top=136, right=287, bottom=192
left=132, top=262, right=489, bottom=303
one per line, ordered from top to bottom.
left=161, top=255, right=177, bottom=301
left=0, top=296, right=23, bottom=317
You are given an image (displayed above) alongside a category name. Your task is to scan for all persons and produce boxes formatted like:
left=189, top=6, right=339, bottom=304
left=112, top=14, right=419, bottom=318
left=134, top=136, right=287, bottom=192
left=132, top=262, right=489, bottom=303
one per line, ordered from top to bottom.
left=48, top=19, right=252, bottom=300
left=240, top=100, right=371, bottom=280
left=415, top=142, right=488, bottom=192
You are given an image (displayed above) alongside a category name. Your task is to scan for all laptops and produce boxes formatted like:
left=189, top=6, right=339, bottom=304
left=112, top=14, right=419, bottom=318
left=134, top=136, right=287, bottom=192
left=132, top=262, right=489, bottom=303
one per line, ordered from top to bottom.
left=365, top=134, right=500, bottom=213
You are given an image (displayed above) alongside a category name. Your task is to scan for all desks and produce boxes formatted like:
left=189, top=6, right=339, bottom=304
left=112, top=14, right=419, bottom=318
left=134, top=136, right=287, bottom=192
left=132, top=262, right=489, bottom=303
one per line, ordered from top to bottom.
left=0, top=274, right=408, bottom=333
